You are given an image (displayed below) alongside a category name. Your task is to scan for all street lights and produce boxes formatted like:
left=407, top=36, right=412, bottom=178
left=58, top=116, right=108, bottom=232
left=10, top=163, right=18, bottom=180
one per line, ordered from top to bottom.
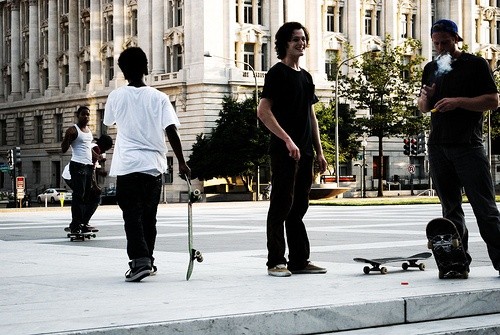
left=335, top=44, right=381, bottom=198
left=203, top=51, right=261, bottom=201
left=360, top=140, right=367, bottom=198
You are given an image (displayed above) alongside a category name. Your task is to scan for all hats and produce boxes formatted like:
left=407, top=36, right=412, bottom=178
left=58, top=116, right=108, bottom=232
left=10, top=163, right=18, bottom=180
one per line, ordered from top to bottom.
left=431, top=20, right=464, bottom=43
left=94, top=135, right=113, bottom=145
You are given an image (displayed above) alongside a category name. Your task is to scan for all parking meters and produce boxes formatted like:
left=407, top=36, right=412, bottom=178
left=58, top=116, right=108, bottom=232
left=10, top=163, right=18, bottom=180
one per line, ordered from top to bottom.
left=15, top=176, right=26, bottom=208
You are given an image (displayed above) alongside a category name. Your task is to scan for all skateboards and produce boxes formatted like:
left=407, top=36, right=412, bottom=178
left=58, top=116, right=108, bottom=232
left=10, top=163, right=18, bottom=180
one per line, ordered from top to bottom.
left=184, top=173, right=204, bottom=280
left=426, top=218, right=470, bottom=280
left=67, top=232, right=92, bottom=241
left=353, top=252, right=432, bottom=274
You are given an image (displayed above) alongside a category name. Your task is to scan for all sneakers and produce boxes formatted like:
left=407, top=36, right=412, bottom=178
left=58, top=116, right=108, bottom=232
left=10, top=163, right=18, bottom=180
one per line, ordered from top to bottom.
left=81, top=223, right=95, bottom=230
left=71, top=227, right=81, bottom=233
left=288, top=262, right=326, bottom=274
left=268, top=264, right=292, bottom=277
left=125, top=265, right=152, bottom=281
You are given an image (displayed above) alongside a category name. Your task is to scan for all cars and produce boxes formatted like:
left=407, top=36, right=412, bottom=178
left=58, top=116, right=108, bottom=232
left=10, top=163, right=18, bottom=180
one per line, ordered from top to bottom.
left=37, top=188, right=72, bottom=204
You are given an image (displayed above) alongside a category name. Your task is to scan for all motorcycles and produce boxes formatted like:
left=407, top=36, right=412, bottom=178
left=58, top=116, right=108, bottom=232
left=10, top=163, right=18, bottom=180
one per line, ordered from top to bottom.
left=262, top=189, right=271, bottom=200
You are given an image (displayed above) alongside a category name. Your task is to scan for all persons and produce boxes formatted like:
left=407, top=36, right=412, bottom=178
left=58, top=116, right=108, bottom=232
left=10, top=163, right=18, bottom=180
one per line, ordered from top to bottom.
left=417, top=20, right=500, bottom=279
left=255, top=22, right=328, bottom=277
left=102, top=46, right=193, bottom=284
left=60, top=106, right=106, bottom=232
left=62, top=134, right=113, bottom=228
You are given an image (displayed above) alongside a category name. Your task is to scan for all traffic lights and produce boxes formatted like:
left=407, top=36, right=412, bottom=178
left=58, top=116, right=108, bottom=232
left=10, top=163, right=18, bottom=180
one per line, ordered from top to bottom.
left=418, top=133, right=426, bottom=153
left=404, top=138, right=410, bottom=156
left=411, top=138, right=418, bottom=155
left=15, top=147, right=22, bottom=167
left=7, top=149, right=14, bottom=171
